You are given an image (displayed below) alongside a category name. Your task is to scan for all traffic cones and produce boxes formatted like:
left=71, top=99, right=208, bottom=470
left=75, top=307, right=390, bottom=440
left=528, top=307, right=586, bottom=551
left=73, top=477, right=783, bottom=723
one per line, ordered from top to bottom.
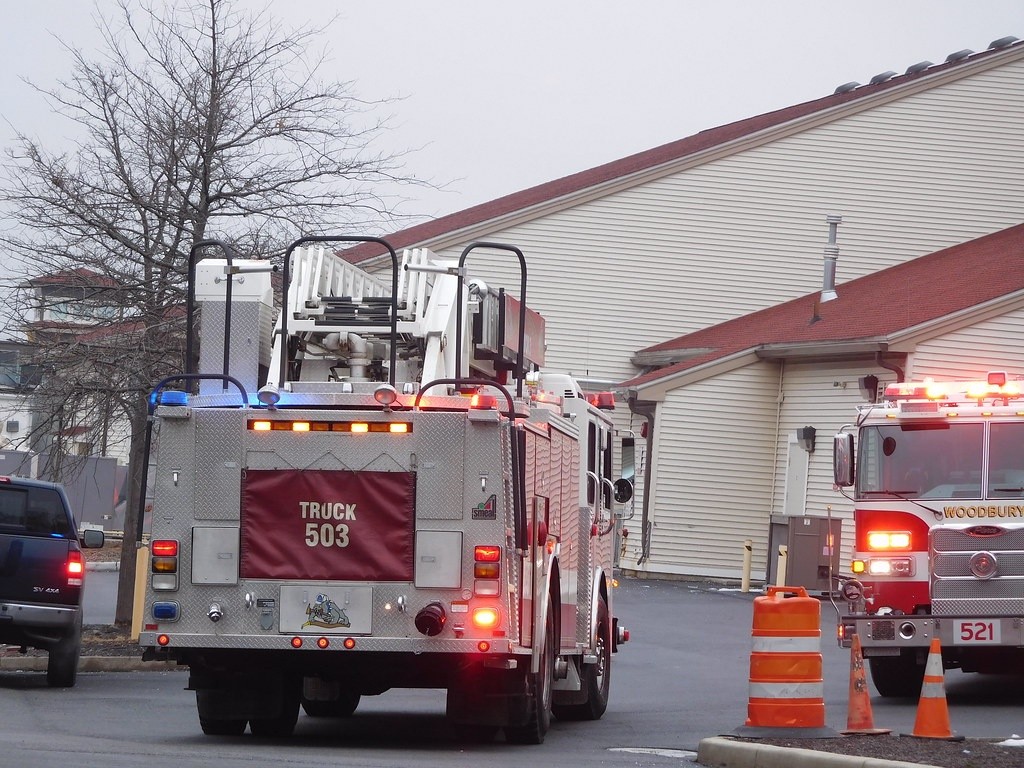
left=836, top=635, right=894, bottom=737
left=899, top=637, right=966, bottom=743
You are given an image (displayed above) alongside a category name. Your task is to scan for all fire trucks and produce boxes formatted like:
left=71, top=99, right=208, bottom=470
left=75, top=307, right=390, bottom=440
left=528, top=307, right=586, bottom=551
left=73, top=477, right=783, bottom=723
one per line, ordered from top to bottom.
left=137, top=232, right=635, bottom=748
left=830, top=371, right=1024, bottom=700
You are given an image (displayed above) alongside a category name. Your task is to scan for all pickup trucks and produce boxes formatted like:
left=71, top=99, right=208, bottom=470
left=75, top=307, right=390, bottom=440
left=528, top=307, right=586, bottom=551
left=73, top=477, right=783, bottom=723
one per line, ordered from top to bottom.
left=0, top=474, right=105, bottom=689
